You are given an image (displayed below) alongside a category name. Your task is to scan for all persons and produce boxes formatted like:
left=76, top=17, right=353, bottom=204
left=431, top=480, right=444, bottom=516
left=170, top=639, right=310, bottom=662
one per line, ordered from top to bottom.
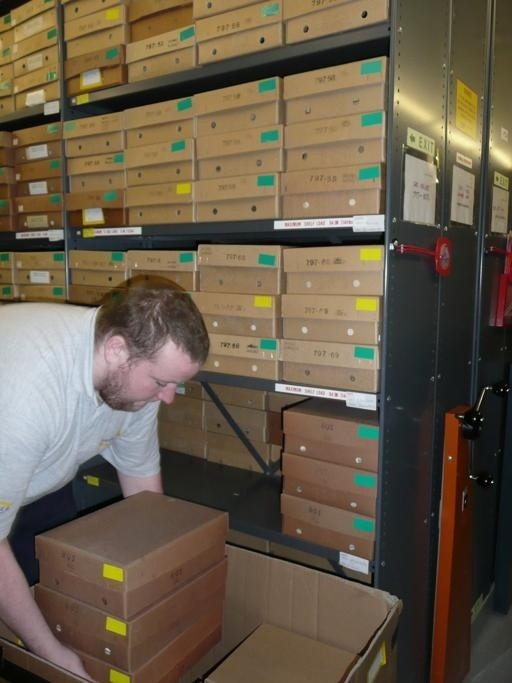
left=0, top=273, right=212, bottom=683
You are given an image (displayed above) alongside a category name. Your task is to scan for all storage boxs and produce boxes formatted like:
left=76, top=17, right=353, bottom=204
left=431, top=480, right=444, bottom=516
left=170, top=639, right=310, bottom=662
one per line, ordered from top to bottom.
left=0, top=0, right=405, bottom=683
